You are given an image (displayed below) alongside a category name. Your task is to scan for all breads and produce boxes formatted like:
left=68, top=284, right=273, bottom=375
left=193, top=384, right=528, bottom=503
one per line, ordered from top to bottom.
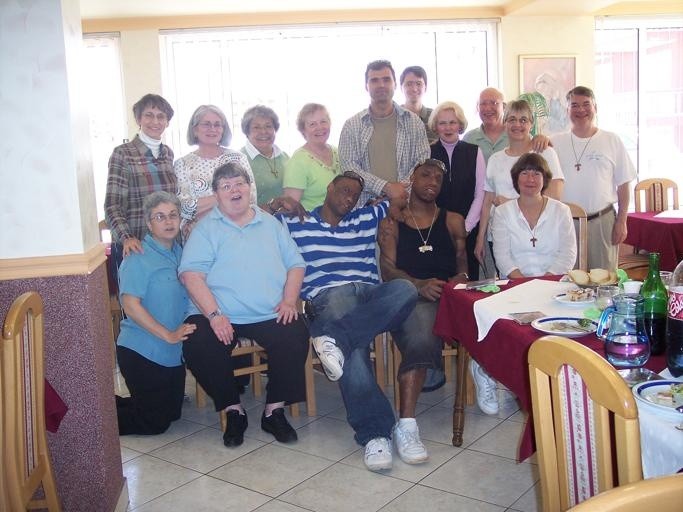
left=568, top=268, right=618, bottom=285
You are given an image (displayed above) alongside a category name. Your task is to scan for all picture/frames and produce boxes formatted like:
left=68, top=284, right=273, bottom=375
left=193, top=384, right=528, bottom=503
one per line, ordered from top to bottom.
left=518, top=55, right=579, bottom=140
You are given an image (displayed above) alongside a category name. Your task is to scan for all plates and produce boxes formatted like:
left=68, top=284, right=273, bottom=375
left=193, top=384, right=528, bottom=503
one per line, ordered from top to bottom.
left=549, top=293, right=598, bottom=306
left=528, top=316, right=598, bottom=338
left=630, top=379, right=683, bottom=414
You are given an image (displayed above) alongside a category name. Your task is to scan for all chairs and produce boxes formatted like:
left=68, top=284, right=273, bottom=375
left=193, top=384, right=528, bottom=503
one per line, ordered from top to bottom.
left=618, top=179, right=679, bottom=281
left=565, top=203, right=588, bottom=271
left=102, top=221, right=123, bottom=342
left=0, top=288, right=63, bottom=511
left=196, top=308, right=475, bottom=433
left=524, top=335, right=645, bottom=511
left=565, top=471, right=683, bottom=512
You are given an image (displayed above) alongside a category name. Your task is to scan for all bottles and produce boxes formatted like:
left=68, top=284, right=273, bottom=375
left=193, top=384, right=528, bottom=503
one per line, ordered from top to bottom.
left=636, top=251, right=668, bottom=356
left=665, top=260, right=683, bottom=378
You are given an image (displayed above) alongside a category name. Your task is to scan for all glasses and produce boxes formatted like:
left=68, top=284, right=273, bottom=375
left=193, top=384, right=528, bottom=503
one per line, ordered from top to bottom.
left=477, top=100, right=505, bottom=108
left=505, top=116, right=532, bottom=125
left=412, top=157, right=448, bottom=176
left=336, top=169, right=366, bottom=189
left=219, top=180, right=248, bottom=193
left=148, top=209, right=181, bottom=223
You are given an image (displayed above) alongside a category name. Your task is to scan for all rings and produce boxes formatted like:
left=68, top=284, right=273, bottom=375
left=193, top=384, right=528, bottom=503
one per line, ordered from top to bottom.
left=536, top=141, right=539, bottom=144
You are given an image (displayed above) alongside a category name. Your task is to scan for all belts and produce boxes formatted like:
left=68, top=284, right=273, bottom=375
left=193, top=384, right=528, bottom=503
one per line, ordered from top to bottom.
left=571, top=203, right=616, bottom=224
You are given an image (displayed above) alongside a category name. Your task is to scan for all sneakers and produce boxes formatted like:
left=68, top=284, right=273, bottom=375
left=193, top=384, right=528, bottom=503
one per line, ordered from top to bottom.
left=311, top=333, right=346, bottom=382
left=470, top=358, right=500, bottom=416
left=259, top=361, right=271, bottom=378
left=241, top=384, right=249, bottom=393
left=183, top=394, right=193, bottom=404
left=259, top=405, right=299, bottom=444
left=222, top=406, right=249, bottom=449
left=392, top=416, right=431, bottom=466
left=362, top=435, right=394, bottom=473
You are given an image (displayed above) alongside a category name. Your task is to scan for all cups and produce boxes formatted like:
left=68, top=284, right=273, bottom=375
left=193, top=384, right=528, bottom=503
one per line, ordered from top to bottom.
left=659, top=271, right=674, bottom=288
left=623, top=281, right=642, bottom=294
left=596, top=285, right=620, bottom=311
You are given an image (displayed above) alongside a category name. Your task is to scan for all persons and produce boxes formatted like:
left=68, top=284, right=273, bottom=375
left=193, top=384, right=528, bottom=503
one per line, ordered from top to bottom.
left=337, top=59, right=431, bottom=209
left=396, top=65, right=437, bottom=143
left=549, top=86, right=638, bottom=274
left=461, top=87, right=554, bottom=280
left=103, top=92, right=177, bottom=285
left=472, top=99, right=565, bottom=279
left=427, top=101, right=487, bottom=281
left=171, top=103, right=256, bottom=247
left=281, top=103, right=343, bottom=211
left=239, top=105, right=288, bottom=207
left=490, top=152, right=578, bottom=279
left=378, top=157, right=499, bottom=468
left=178, top=161, right=310, bottom=447
left=256, top=170, right=419, bottom=473
left=109, top=190, right=197, bottom=436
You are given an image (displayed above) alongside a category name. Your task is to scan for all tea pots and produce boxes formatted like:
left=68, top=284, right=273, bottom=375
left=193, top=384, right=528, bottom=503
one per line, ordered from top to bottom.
left=596, top=292, right=652, bottom=368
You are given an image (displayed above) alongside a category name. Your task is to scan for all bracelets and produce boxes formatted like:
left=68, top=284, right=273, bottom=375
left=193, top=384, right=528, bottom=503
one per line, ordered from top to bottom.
left=266, top=197, right=281, bottom=213
left=456, top=272, right=469, bottom=280
left=206, top=308, right=221, bottom=322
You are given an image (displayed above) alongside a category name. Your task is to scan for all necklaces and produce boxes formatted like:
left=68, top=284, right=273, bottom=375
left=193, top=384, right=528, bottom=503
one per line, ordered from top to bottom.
left=568, top=128, right=596, bottom=170
left=301, top=145, right=341, bottom=173
left=259, top=149, right=279, bottom=178
left=505, top=149, right=531, bottom=157
left=515, top=194, right=546, bottom=249
left=405, top=203, right=437, bottom=254
